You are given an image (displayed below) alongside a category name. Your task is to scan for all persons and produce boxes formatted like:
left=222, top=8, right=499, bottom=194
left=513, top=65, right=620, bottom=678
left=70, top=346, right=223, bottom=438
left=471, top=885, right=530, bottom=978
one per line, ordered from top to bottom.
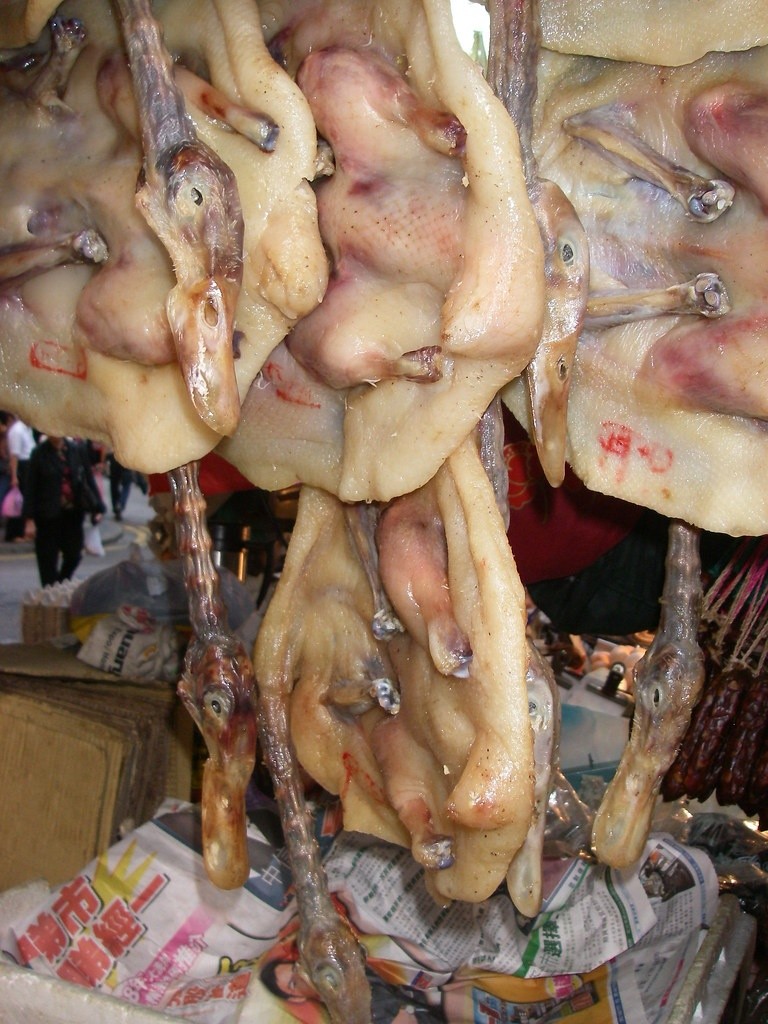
left=0, top=410, right=147, bottom=588
left=261, top=960, right=448, bottom=1024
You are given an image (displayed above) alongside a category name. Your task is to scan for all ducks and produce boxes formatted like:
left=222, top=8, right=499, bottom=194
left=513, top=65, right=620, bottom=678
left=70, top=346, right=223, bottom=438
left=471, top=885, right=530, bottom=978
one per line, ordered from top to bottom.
left=1, top=0, right=768, bottom=1023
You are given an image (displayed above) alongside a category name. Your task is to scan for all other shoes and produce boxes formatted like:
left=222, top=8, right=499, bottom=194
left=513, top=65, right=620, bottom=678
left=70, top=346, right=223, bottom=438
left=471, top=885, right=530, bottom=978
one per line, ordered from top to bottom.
left=114, top=508, right=121, bottom=520
left=14, top=536, right=33, bottom=542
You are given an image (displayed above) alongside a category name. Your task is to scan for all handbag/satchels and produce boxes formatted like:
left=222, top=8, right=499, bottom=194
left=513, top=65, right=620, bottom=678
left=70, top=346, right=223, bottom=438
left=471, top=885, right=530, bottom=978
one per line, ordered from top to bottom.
left=136, top=474, right=148, bottom=494
left=1, top=486, right=23, bottom=516
left=84, top=523, right=106, bottom=557
left=69, top=555, right=262, bottom=686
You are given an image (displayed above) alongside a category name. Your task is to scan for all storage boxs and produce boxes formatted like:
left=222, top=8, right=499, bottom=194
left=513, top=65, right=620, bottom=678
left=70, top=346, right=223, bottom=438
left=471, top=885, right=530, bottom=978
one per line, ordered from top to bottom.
left=18, top=604, right=72, bottom=646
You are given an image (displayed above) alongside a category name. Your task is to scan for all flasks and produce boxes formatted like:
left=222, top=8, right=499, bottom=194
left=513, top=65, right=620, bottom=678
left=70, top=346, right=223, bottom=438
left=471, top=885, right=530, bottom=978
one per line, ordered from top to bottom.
left=205, top=521, right=276, bottom=613
